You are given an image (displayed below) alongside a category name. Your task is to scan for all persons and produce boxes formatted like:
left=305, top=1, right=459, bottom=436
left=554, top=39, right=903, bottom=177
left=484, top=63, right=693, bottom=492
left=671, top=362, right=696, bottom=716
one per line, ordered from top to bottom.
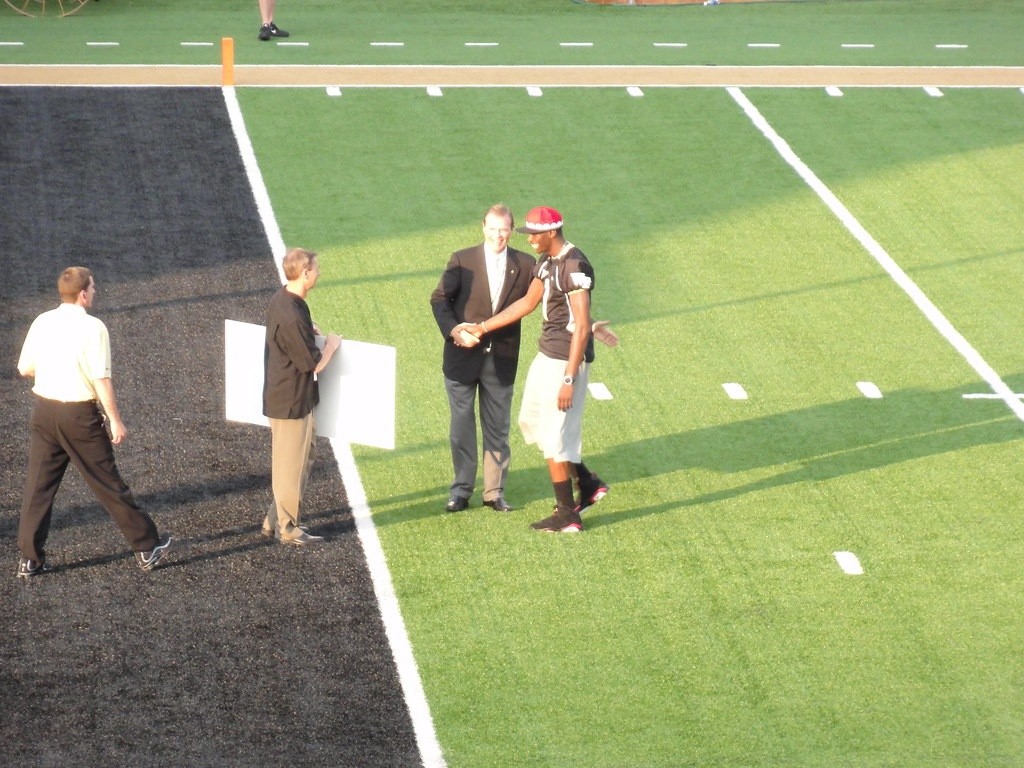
left=258, top=0, right=289, bottom=41
left=430, top=205, right=619, bottom=531
left=261, top=247, right=343, bottom=544
left=16, top=267, right=175, bottom=576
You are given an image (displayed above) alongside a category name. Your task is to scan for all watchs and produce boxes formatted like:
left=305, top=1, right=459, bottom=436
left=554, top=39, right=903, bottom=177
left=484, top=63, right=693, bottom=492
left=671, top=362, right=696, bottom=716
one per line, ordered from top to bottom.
left=563, top=372, right=575, bottom=386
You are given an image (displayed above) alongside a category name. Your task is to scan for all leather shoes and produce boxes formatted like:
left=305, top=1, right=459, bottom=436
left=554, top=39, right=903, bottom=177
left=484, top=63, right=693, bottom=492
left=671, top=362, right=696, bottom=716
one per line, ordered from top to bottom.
left=446, top=495, right=468, bottom=512
left=483, top=498, right=512, bottom=512
left=260, top=524, right=310, bottom=540
left=275, top=532, right=324, bottom=544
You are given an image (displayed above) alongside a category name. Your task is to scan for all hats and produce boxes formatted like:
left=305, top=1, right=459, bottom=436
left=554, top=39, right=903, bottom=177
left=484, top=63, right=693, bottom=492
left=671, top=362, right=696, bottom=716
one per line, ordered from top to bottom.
left=514, top=205, right=563, bottom=234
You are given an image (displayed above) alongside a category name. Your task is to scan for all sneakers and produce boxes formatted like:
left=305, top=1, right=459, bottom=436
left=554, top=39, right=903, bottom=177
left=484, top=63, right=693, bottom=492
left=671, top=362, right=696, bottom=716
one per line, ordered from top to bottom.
left=258, top=23, right=271, bottom=39
left=269, top=23, right=289, bottom=37
left=574, top=472, right=607, bottom=511
left=529, top=504, right=582, bottom=532
left=134, top=532, right=173, bottom=572
left=16, top=557, right=41, bottom=579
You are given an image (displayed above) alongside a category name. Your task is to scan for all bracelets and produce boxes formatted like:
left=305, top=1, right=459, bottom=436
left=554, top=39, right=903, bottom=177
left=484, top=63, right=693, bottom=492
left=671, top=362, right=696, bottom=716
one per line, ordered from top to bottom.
left=482, top=321, right=490, bottom=332
